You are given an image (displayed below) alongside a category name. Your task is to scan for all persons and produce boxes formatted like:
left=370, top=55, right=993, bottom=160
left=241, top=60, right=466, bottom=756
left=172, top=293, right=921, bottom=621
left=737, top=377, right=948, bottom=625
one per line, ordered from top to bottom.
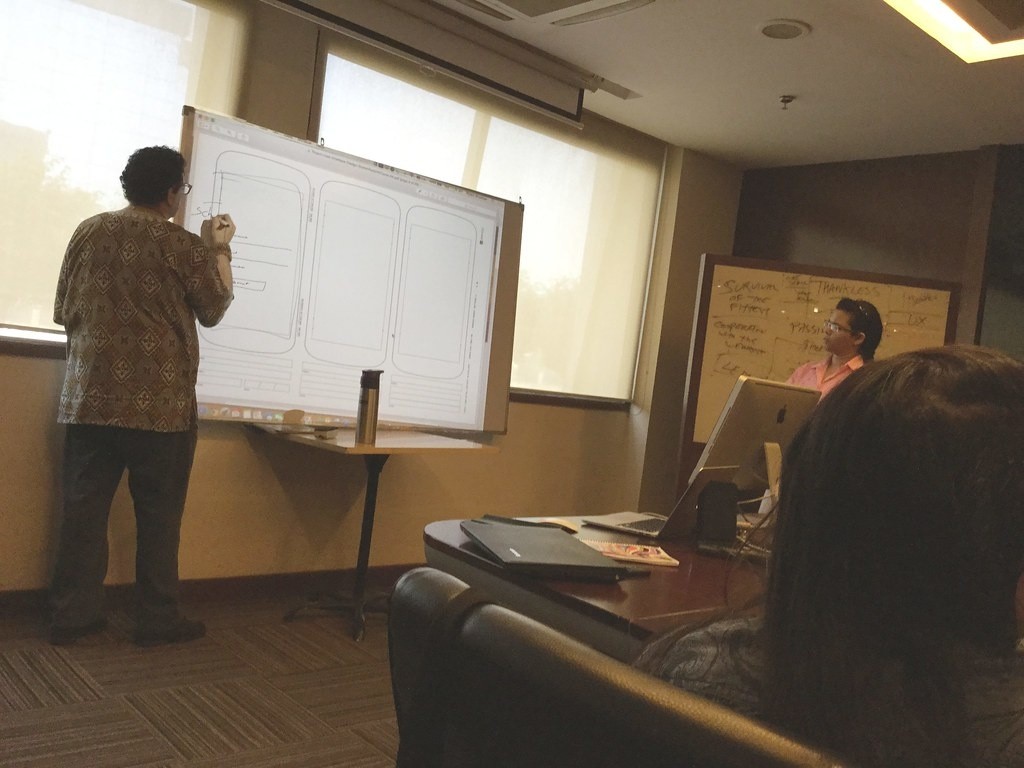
left=49, top=146, right=237, bottom=640
left=783, top=297, right=884, bottom=402
left=626, top=343, right=1024, bottom=768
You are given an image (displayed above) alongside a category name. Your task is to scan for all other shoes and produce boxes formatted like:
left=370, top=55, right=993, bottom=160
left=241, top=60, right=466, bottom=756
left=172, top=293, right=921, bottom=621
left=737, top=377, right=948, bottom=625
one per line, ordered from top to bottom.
left=46, top=610, right=108, bottom=644
left=134, top=611, right=205, bottom=647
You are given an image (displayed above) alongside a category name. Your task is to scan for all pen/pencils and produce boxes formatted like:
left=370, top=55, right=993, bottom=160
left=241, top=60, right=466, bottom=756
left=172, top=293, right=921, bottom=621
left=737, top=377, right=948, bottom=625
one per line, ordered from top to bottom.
left=212, top=215, right=229, bottom=228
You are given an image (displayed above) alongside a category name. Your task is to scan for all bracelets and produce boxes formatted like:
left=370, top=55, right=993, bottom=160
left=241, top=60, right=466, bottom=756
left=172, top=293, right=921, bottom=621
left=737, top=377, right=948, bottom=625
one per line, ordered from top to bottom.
left=213, top=241, right=233, bottom=260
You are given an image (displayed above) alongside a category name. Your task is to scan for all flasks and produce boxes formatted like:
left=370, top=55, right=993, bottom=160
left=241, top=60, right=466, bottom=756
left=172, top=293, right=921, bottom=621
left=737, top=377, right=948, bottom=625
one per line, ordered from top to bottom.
left=355, top=369, right=384, bottom=448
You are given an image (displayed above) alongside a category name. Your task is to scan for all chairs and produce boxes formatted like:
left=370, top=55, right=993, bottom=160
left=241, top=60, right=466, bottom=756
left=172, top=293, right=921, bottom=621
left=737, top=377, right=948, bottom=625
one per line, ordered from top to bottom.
left=386, top=568, right=853, bottom=768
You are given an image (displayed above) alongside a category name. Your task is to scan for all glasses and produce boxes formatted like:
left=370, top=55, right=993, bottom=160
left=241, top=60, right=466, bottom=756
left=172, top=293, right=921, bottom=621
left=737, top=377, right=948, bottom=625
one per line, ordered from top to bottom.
left=175, top=183, right=191, bottom=195
left=836, top=299, right=868, bottom=321
left=822, top=320, right=861, bottom=336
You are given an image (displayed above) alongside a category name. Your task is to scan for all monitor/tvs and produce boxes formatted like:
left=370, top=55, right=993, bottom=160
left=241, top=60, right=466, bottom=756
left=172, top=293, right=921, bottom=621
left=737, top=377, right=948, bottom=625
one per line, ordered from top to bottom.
left=688, top=376, right=823, bottom=531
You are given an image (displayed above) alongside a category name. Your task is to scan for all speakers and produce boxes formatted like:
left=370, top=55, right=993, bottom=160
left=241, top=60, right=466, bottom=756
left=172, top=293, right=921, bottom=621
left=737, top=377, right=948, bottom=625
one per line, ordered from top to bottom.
left=696, top=481, right=739, bottom=545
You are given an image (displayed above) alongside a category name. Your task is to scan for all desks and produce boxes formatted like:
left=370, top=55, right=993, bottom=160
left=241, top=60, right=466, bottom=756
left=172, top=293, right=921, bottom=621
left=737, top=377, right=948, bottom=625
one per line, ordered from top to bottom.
left=244, top=420, right=502, bottom=643
left=422, top=511, right=773, bottom=670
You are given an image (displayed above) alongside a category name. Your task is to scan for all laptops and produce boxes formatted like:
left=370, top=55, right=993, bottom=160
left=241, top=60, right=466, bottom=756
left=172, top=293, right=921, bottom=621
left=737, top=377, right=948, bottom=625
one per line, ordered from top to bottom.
left=583, top=465, right=742, bottom=539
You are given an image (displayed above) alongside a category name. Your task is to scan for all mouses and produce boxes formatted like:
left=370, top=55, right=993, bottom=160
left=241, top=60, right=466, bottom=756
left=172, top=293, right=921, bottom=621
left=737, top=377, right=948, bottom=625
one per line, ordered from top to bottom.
left=539, top=518, right=577, bottom=536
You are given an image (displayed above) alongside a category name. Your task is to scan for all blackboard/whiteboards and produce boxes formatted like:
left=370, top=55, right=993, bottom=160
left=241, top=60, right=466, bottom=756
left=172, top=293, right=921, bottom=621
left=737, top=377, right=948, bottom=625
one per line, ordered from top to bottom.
left=181, top=108, right=526, bottom=434
left=677, top=250, right=963, bottom=484
